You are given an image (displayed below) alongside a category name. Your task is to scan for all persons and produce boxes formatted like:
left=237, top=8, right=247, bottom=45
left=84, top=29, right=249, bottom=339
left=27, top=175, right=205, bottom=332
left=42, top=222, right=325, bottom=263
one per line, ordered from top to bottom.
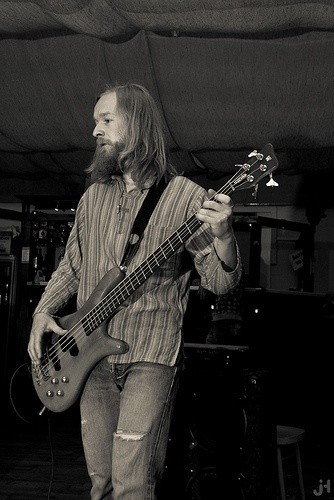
left=26, top=84, right=244, bottom=499
left=192, top=278, right=245, bottom=347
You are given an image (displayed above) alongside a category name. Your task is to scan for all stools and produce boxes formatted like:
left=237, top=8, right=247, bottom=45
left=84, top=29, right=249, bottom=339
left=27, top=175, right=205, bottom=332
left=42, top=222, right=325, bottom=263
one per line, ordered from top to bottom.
left=262, top=423, right=309, bottom=500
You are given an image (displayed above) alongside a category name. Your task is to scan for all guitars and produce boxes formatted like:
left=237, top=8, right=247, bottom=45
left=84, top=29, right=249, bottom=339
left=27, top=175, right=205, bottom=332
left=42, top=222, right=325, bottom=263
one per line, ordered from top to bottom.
left=30, top=142, right=280, bottom=413
